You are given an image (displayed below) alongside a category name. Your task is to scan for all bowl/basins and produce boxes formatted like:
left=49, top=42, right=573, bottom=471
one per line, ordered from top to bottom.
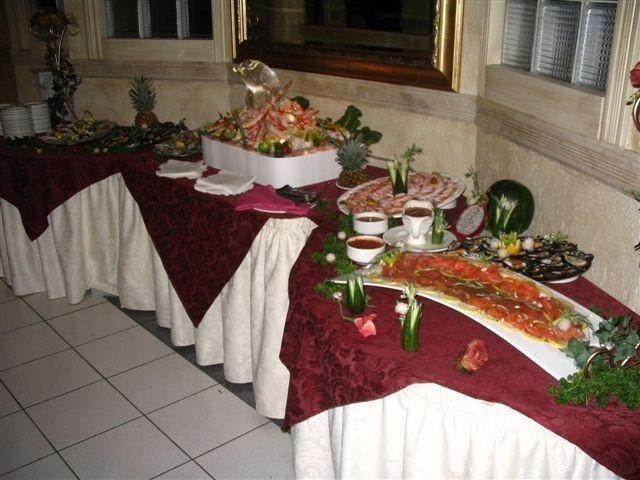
left=353, top=213, right=388, bottom=234
left=345, top=235, right=386, bottom=262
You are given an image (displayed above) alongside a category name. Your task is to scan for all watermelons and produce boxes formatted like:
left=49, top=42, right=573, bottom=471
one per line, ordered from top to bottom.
left=486, top=178, right=535, bottom=238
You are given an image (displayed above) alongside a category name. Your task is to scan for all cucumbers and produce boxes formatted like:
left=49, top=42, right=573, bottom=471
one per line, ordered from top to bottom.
left=494, top=192, right=517, bottom=236
left=431, top=212, right=445, bottom=245
left=346, top=273, right=365, bottom=315
left=401, top=304, right=422, bottom=353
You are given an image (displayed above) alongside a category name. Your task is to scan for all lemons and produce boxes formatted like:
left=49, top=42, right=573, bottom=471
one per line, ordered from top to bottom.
left=259, top=139, right=270, bottom=153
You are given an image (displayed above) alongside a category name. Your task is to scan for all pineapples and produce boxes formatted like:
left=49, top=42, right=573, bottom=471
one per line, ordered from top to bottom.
left=126, top=74, right=158, bottom=127
left=330, top=126, right=375, bottom=189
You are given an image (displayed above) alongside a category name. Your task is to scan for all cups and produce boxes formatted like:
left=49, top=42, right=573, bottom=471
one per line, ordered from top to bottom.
left=401, top=200, right=434, bottom=245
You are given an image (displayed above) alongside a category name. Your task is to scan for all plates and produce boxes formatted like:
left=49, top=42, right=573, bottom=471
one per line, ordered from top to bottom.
left=0, top=101, right=52, bottom=139
left=154, top=143, right=191, bottom=158
left=337, top=173, right=465, bottom=218
left=336, top=184, right=351, bottom=190
left=255, top=204, right=316, bottom=214
left=383, top=225, right=457, bottom=252
left=324, top=255, right=640, bottom=390
left=547, top=275, right=579, bottom=283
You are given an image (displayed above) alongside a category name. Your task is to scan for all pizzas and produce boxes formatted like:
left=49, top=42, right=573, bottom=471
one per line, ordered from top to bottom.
left=347, top=172, right=453, bottom=214
